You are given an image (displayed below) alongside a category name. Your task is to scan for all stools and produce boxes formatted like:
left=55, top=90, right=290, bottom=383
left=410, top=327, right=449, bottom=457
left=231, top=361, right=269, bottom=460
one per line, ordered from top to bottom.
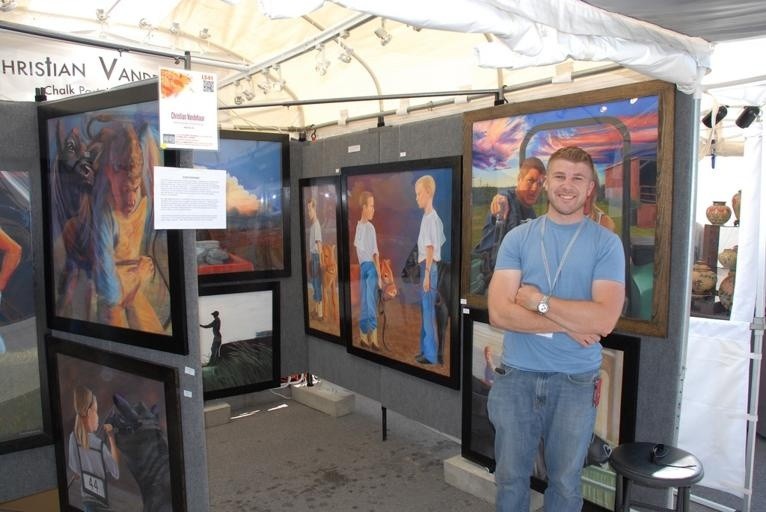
left=608, top=442, right=704, bottom=512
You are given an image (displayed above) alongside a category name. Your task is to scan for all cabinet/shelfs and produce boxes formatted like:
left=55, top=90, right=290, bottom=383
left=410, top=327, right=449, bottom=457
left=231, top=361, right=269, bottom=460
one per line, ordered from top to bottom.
left=689, top=224, right=740, bottom=320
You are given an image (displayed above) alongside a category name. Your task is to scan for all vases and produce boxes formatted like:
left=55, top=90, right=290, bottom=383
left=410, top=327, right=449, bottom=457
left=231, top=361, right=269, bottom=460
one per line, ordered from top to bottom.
left=732, top=190, right=741, bottom=219
left=705, top=200, right=732, bottom=225
left=717, top=249, right=737, bottom=268
left=692, top=261, right=717, bottom=294
left=717, top=271, right=735, bottom=311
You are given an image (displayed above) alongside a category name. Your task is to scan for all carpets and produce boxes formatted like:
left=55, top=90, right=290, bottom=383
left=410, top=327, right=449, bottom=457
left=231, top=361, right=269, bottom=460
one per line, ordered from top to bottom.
left=0, top=443, right=59, bottom=505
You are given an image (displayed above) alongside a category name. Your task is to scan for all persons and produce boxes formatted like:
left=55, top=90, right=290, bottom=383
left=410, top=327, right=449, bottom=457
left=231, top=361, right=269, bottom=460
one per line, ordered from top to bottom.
left=88, top=120, right=167, bottom=332
left=486, top=146, right=626, bottom=512
left=471, top=157, right=546, bottom=295
left=583, top=168, right=617, bottom=232
left=415, top=175, right=446, bottom=364
left=354, top=191, right=384, bottom=351
left=308, top=198, right=324, bottom=321
left=1, top=230, right=23, bottom=354
left=200, top=311, right=223, bottom=365
left=68, top=386, right=122, bottom=512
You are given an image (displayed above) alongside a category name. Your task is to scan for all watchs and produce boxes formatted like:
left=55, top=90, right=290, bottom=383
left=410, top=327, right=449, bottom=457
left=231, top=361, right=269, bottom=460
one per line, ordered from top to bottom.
left=537, top=295, right=551, bottom=316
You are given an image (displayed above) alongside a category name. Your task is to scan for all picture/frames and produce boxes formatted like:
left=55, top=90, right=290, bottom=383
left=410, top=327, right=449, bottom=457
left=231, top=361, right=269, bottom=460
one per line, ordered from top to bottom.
left=459, top=76, right=675, bottom=338
left=35, top=81, right=189, bottom=356
left=193, top=129, right=292, bottom=280
left=341, top=156, right=461, bottom=390
left=298, top=175, right=347, bottom=346
left=198, top=279, right=281, bottom=400
left=460, top=309, right=640, bottom=512
left=43, top=337, right=187, bottom=512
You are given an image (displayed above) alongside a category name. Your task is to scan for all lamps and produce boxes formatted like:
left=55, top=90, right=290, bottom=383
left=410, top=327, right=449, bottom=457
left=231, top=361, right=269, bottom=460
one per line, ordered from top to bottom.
left=0, top=0, right=212, bottom=42
left=217, top=7, right=426, bottom=106
left=702, top=105, right=727, bottom=128
left=735, top=105, right=759, bottom=128
